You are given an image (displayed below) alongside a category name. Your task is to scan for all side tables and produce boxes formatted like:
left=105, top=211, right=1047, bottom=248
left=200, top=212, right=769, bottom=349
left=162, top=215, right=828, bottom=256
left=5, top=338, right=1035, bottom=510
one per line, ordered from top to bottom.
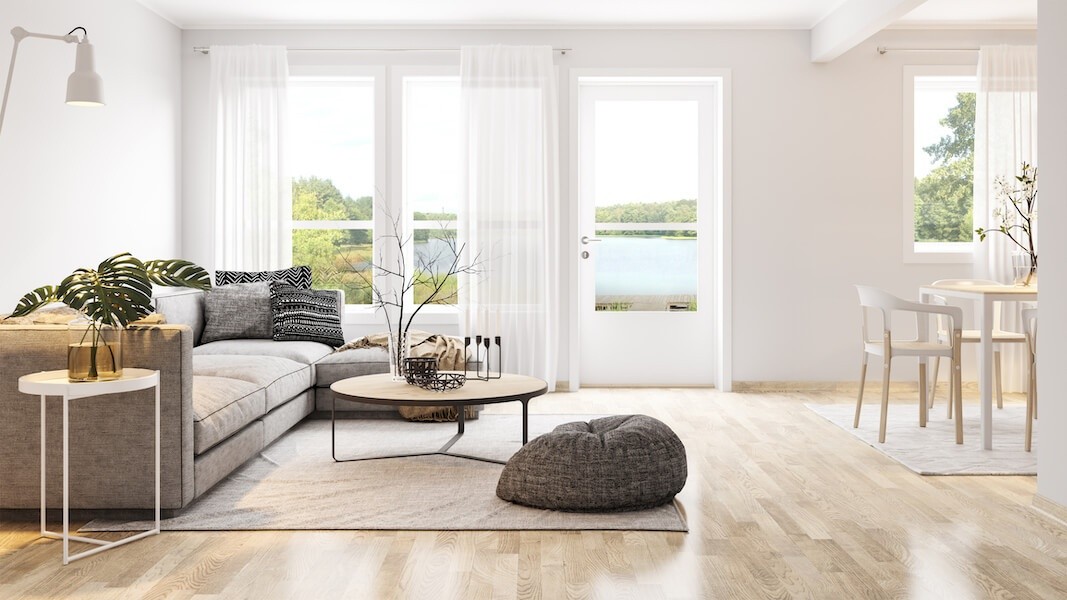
left=18, top=366, right=165, bottom=566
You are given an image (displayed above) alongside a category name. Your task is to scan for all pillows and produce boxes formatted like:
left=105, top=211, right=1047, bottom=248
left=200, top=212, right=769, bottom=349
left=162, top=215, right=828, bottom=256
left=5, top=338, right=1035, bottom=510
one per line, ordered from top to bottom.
left=215, top=266, right=314, bottom=294
left=200, top=280, right=286, bottom=343
left=272, top=281, right=344, bottom=347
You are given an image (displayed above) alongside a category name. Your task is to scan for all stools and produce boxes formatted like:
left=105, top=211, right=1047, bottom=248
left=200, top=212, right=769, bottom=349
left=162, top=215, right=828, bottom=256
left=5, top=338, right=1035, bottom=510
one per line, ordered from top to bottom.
left=314, top=334, right=486, bottom=423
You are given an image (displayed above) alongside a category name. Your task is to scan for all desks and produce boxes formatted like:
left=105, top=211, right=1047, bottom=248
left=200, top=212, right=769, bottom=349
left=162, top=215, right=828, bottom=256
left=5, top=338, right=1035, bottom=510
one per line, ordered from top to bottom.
left=919, top=283, right=1039, bottom=449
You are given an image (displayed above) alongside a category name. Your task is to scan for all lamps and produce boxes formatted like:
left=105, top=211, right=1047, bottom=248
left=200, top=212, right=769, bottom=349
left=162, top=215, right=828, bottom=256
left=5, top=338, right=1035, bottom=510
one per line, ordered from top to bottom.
left=0, top=25, right=106, bottom=134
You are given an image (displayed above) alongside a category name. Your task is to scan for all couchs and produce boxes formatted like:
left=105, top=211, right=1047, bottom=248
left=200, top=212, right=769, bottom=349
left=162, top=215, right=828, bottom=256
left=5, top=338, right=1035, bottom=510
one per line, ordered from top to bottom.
left=0, top=283, right=348, bottom=515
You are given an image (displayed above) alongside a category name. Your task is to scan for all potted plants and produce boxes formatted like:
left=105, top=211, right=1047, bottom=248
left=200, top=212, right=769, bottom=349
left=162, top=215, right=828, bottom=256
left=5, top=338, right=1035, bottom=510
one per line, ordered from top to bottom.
left=5, top=250, right=215, bottom=383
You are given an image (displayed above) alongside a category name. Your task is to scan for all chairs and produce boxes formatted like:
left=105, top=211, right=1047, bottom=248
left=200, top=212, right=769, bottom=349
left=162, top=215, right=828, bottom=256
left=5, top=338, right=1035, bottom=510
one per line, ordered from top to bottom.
left=853, top=274, right=1040, bottom=442
left=495, top=410, right=688, bottom=510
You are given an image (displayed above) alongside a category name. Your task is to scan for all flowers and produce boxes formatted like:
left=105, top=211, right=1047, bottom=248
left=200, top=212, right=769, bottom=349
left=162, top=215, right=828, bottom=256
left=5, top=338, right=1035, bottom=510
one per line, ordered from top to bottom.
left=973, top=160, right=1042, bottom=250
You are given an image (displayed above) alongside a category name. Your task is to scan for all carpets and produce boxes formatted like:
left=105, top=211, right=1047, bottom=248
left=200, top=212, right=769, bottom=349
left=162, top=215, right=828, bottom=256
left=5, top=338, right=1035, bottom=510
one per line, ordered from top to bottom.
left=76, top=392, right=691, bottom=534
left=803, top=403, right=1041, bottom=475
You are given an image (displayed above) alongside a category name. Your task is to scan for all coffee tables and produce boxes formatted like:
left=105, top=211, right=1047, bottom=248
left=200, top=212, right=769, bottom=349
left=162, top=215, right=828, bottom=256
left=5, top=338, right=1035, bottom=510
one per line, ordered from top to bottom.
left=331, top=371, right=549, bottom=464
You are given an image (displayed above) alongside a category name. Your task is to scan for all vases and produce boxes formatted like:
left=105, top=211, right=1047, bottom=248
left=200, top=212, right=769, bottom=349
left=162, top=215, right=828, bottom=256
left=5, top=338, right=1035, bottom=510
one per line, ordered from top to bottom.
left=1010, top=243, right=1041, bottom=286
left=390, top=329, right=413, bottom=380
